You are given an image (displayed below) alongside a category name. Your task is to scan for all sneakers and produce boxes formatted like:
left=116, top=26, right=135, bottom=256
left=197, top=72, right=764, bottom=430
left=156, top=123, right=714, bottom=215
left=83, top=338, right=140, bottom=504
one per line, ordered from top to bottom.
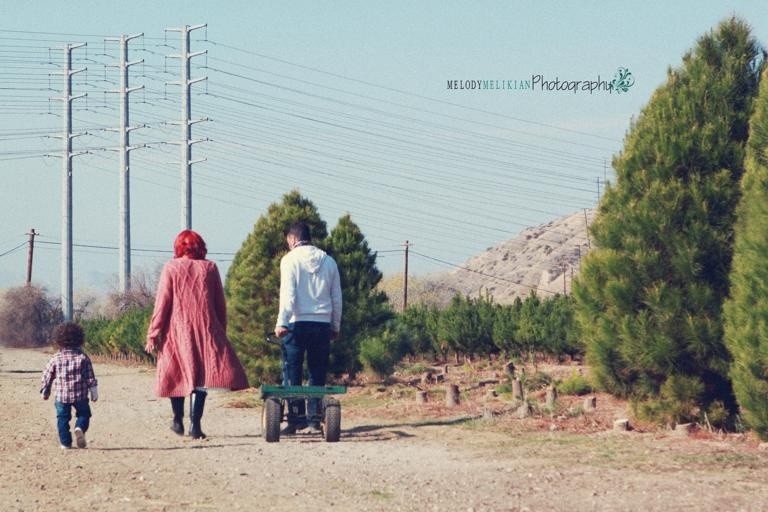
left=280, top=421, right=319, bottom=434
left=60, top=427, right=88, bottom=450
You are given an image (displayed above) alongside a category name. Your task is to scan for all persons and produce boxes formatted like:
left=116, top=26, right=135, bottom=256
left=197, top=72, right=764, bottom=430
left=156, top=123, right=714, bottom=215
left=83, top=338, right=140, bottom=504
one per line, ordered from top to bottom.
left=274, top=221, right=343, bottom=435
left=143, top=229, right=229, bottom=439
left=39, top=320, right=98, bottom=451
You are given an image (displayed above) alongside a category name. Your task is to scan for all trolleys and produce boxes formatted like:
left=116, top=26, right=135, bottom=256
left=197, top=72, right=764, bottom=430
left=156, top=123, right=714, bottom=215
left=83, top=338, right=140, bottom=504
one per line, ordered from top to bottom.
left=260, top=331, right=346, bottom=440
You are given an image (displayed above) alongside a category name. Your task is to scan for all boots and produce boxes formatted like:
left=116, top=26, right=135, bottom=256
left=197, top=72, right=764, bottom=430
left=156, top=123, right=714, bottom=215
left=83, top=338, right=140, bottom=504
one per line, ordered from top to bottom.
left=190, top=390, right=206, bottom=438
left=169, top=397, right=185, bottom=435
